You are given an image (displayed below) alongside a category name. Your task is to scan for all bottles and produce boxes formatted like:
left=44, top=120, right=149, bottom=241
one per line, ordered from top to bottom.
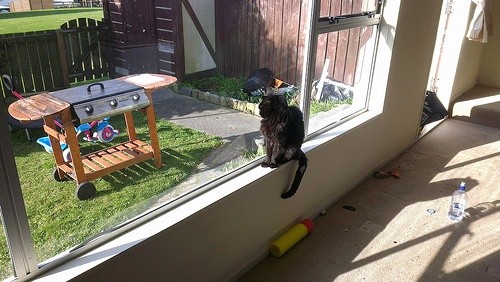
left=448, top=182, right=469, bottom=222
left=271, top=218, right=315, bottom=257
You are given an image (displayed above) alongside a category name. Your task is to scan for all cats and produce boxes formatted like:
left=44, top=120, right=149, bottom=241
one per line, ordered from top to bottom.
left=258, top=93, right=309, bottom=200
